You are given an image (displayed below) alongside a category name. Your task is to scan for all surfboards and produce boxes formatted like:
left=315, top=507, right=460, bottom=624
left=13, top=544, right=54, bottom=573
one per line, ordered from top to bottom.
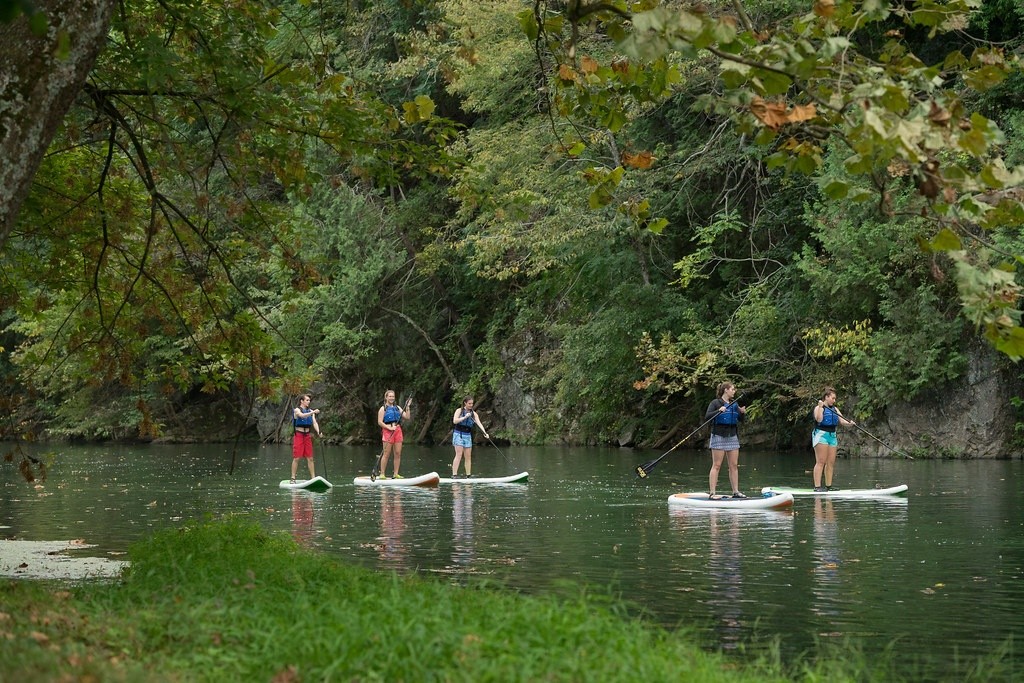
left=354, top=471, right=440, bottom=486
left=441, top=471, right=529, bottom=485
left=279, top=475, right=333, bottom=490
left=762, top=483, right=908, bottom=497
left=666, top=490, right=794, bottom=511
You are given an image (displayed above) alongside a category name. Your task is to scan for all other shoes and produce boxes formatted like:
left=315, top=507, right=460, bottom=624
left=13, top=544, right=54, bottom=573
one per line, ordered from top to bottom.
left=392, top=473, right=404, bottom=479
left=379, top=474, right=386, bottom=480
left=452, top=475, right=458, bottom=479
left=466, top=475, right=475, bottom=478
left=290, top=477, right=296, bottom=484
left=814, top=486, right=828, bottom=492
left=826, top=486, right=840, bottom=490
left=731, top=491, right=746, bottom=498
left=709, top=493, right=728, bottom=500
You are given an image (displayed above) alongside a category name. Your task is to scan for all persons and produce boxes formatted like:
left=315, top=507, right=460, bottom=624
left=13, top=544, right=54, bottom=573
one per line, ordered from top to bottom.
left=705, top=381, right=747, bottom=500
left=811, top=389, right=856, bottom=492
left=378, top=390, right=413, bottom=480
left=289, top=394, right=324, bottom=484
left=450, top=396, right=490, bottom=479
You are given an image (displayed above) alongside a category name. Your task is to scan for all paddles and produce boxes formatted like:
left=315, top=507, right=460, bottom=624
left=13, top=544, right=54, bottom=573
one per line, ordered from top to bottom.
left=371, top=390, right=414, bottom=481
left=633, top=393, right=744, bottom=478
left=806, top=395, right=914, bottom=461
left=317, top=414, right=328, bottom=481
left=468, top=415, right=518, bottom=471
